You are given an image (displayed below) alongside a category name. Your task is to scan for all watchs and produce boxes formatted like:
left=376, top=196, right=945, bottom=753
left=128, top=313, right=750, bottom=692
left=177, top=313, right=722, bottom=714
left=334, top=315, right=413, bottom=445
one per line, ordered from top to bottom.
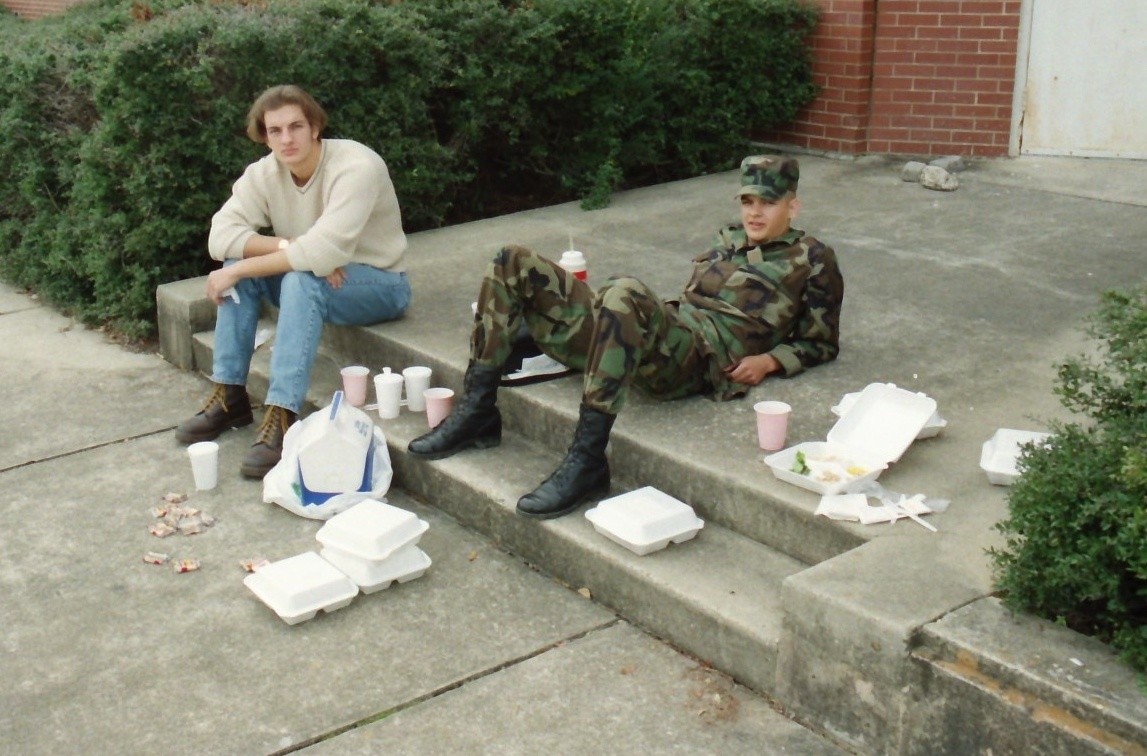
left=278, top=236, right=292, bottom=251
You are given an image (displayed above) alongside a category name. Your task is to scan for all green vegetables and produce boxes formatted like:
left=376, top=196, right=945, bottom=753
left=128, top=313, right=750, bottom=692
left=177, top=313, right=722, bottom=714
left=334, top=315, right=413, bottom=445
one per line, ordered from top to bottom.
left=789, top=450, right=811, bottom=475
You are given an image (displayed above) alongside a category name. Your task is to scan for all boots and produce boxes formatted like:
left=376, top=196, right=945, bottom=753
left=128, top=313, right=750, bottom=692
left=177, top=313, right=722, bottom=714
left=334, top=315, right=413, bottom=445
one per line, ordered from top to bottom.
left=407, top=359, right=503, bottom=459
left=516, top=404, right=616, bottom=517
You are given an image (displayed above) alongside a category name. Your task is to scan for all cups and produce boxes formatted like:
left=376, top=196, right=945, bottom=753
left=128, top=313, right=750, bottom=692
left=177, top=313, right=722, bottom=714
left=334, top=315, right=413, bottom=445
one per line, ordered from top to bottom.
left=340, top=365, right=370, bottom=407
left=404, top=365, right=432, bottom=412
left=374, top=372, right=404, bottom=420
left=424, top=388, right=455, bottom=427
left=754, top=401, right=791, bottom=450
left=187, top=440, right=219, bottom=490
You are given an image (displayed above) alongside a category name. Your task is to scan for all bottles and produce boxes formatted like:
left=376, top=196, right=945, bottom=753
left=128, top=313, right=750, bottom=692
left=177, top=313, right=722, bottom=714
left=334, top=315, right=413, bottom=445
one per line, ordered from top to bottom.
left=560, top=251, right=586, bottom=282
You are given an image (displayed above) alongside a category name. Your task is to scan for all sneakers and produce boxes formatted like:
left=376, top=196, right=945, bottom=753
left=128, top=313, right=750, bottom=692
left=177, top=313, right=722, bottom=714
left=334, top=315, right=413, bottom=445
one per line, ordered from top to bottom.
left=175, top=383, right=253, bottom=443
left=240, top=405, right=295, bottom=477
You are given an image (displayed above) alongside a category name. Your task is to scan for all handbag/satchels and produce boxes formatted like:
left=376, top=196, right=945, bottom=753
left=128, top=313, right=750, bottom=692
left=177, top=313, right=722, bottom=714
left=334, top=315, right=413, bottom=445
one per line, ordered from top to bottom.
left=259, top=390, right=393, bottom=519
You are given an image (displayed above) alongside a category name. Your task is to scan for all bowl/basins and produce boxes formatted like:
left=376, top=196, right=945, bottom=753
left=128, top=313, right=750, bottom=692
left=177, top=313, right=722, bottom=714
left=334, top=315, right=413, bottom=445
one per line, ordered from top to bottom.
left=765, top=381, right=949, bottom=498
left=980, top=427, right=1062, bottom=486
left=585, top=486, right=704, bottom=557
left=314, top=498, right=431, bottom=566
left=320, top=545, right=433, bottom=596
left=243, top=552, right=360, bottom=625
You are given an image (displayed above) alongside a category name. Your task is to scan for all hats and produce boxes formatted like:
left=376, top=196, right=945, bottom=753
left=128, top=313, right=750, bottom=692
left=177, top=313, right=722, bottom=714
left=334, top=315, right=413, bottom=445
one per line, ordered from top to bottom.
left=733, top=155, right=798, bottom=201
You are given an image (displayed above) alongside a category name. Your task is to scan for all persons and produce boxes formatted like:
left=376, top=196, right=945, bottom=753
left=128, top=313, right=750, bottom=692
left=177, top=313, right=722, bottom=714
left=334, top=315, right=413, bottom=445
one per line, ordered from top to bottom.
left=173, top=85, right=410, bottom=478
left=405, top=154, right=844, bottom=521
